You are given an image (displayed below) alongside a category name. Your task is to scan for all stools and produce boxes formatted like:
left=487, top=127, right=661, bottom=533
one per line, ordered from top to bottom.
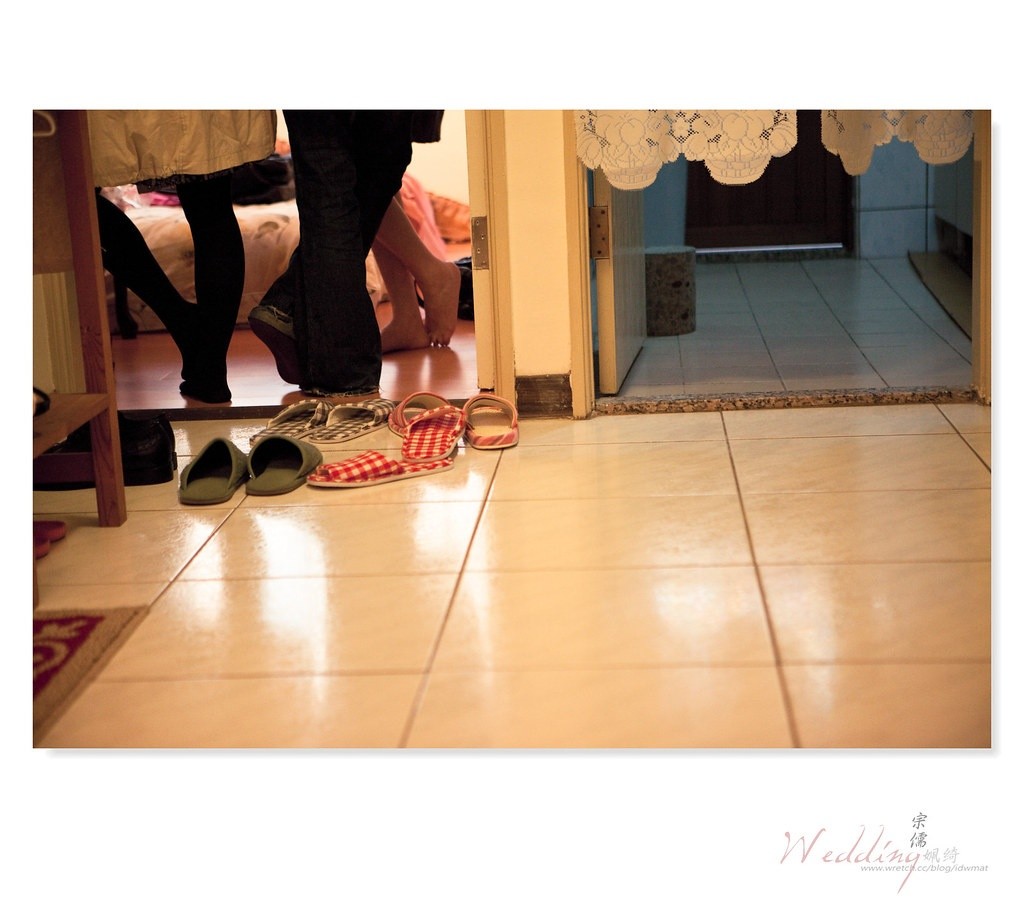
left=643, top=245, right=698, bottom=337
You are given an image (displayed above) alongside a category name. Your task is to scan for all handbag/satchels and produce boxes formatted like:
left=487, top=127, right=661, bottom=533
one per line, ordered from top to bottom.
left=414, top=256, right=474, bottom=321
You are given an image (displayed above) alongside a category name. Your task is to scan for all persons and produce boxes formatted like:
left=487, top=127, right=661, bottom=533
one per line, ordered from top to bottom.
left=87, top=110, right=277, bottom=405
left=250, top=110, right=446, bottom=401
left=369, top=188, right=461, bottom=353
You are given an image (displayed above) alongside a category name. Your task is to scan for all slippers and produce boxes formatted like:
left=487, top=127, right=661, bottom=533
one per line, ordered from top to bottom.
left=179, top=392, right=520, bottom=504
left=33, top=520, right=67, bottom=557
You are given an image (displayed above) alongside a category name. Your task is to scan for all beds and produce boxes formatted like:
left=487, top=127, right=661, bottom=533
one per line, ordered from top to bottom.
left=96, top=167, right=448, bottom=338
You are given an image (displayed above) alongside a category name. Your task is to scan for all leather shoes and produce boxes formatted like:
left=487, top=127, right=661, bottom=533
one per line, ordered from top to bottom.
left=33, top=410, right=177, bottom=490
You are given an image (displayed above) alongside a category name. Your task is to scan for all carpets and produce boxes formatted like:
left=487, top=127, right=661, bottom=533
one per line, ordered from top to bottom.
left=32, top=605, right=150, bottom=748
